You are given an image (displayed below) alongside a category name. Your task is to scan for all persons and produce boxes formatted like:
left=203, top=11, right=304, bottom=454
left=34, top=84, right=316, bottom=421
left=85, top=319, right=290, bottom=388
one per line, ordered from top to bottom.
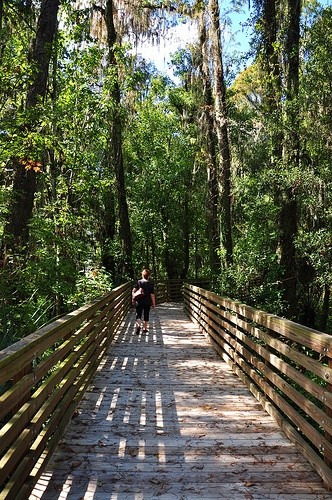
left=132, top=269, right=155, bottom=335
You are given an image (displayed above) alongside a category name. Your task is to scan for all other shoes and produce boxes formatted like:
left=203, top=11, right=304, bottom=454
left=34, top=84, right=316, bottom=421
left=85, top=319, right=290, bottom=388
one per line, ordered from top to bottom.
left=136, top=326, right=141, bottom=335
left=143, top=329, right=149, bottom=333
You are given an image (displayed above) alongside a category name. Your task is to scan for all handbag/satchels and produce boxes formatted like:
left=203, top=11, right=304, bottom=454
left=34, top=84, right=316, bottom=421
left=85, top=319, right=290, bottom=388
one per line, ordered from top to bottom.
left=133, top=288, right=145, bottom=300
